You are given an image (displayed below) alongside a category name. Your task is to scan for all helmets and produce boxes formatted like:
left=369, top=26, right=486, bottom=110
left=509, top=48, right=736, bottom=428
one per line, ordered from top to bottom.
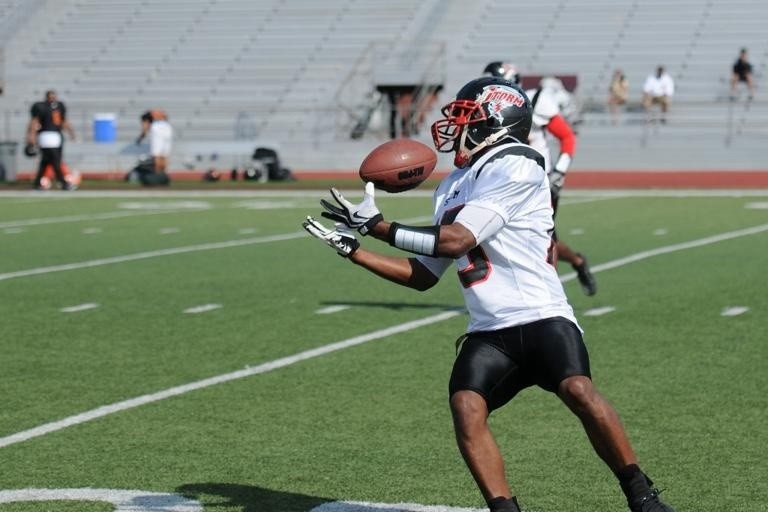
left=482, top=62, right=523, bottom=88
left=430, top=78, right=533, bottom=168
left=24, top=143, right=38, bottom=156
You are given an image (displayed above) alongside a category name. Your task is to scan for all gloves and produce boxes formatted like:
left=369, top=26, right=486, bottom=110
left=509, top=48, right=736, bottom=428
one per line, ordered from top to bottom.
left=549, top=168, right=565, bottom=198
left=320, top=182, right=383, bottom=239
left=302, top=216, right=360, bottom=258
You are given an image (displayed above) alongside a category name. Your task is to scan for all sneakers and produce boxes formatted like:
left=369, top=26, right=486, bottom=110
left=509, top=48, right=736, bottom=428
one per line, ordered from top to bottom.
left=573, top=252, right=597, bottom=295
left=627, top=488, right=677, bottom=512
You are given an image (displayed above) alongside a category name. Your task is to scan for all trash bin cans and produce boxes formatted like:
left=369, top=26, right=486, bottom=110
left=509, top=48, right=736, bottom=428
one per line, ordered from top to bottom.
left=0, top=141, right=18, bottom=183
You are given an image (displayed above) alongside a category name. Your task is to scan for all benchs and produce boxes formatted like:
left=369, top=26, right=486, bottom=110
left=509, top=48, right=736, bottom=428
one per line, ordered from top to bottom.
left=1, top=1, right=767, bottom=151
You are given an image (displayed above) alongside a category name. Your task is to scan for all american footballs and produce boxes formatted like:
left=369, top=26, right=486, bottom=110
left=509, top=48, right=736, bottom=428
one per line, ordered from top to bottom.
left=359, top=139, right=437, bottom=192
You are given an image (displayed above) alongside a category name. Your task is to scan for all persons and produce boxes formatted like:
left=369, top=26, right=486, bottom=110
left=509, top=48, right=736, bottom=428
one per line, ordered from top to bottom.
left=727, top=47, right=755, bottom=100
left=483, top=61, right=598, bottom=298
left=643, top=64, right=674, bottom=125
left=607, top=68, right=630, bottom=115
left=302, top=78, right=676, bottom=511
left=27, top=90, right=80, bottom=191
left=121, top=112, right=173, bottom=184
